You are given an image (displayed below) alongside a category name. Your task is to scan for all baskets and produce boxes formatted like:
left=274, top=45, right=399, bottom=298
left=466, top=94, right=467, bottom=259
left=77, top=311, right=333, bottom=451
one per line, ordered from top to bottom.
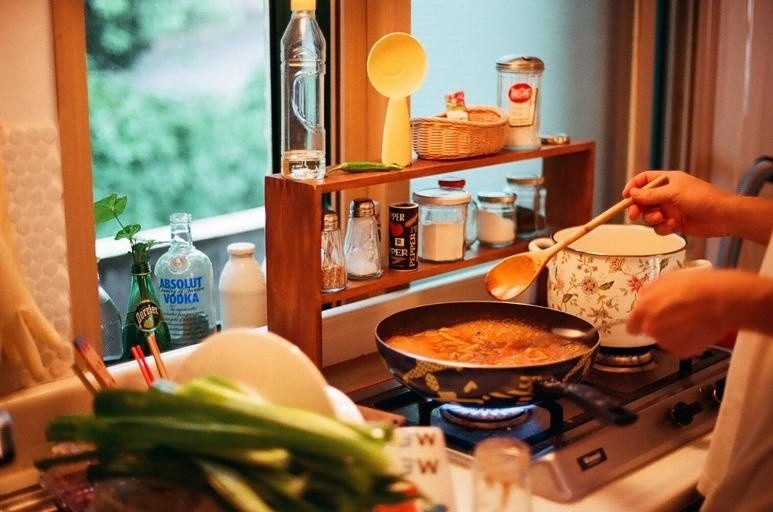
left=409, top=106, right=510, bottom=161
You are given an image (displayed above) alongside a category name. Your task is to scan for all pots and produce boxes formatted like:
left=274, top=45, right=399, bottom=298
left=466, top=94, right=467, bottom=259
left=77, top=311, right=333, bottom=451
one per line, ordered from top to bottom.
left=527, top=221, right=714, bottom=351
left=377, top=298, right=639, bottom=429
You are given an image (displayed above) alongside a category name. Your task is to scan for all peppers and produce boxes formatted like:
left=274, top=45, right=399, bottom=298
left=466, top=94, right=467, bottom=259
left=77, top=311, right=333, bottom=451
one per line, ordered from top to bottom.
left=325, top=161, right=403, bottom=178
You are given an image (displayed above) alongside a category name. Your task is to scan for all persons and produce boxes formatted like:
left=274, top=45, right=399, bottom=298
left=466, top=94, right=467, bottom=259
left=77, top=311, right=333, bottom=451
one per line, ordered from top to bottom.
left=622, top=169, right=773, bottom=512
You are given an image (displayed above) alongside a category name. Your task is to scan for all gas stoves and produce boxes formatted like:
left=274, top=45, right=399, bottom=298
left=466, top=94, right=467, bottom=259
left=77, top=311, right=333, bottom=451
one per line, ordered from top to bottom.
left=348, top=343, right=737, bottom=502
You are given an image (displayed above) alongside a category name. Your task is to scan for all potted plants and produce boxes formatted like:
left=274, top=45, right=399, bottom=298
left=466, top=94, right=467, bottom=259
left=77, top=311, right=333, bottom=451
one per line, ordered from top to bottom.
left=94, top=195, right=173, bottom=361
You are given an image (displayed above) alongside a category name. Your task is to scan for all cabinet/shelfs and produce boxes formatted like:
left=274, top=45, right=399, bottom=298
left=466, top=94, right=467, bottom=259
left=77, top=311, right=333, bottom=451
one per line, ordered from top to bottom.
left=265, top=138, right=598, bottom=396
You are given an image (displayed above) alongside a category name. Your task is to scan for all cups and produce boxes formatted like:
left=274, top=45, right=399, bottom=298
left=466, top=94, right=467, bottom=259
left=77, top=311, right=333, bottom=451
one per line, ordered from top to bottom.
left=388, top=200, right=419, bottom=271
left=467, top=434, right=532, bottom=512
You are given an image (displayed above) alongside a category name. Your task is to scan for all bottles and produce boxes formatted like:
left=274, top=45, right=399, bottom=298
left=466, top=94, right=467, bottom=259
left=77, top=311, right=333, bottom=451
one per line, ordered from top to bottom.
left=280, top=0, right=327, bottom=182
left=492, top=56, right=544, bottom=151
left=411, top=170, right=549, bottom=264
left=322, top=199, right=383, bottom=292
left=154, top=213, right=217, bottom=345
left=220, top=241, right=269, bottom=333
left=96, top=273, right=122, bottom=363
